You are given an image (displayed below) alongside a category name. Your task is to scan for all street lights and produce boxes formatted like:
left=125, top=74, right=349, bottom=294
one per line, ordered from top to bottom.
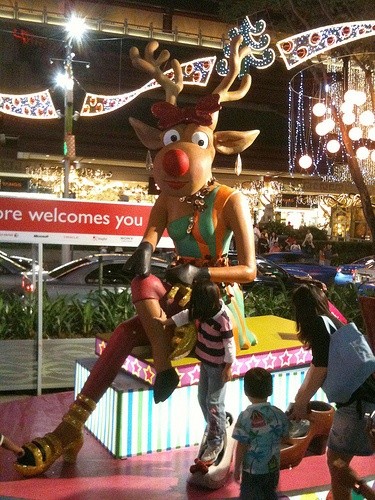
left=48, top=12, right=91, bottom=200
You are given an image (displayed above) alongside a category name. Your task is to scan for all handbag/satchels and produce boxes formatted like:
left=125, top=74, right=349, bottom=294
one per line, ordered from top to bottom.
left=320, top=315, right=375, bottom=404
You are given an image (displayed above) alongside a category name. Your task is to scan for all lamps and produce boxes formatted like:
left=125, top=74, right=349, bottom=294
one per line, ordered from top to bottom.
left=60, top=13, right=91, bottom=61
left=49, top=64, right=81, bottom=199
left=339, top=91, right=366, bottom=113
left=313, top=103, right=326, bottom=116
left=360, top=110, right=375, bottom=126
left=342, top=113, right=356, bottom=125
left=314, top=119, right=335, bottom=135
left=349, top=127, right=363, bottom=142
left=368, top=127, right=375, bottom=141
left=327, top=140, right=341, bottom=153
left=357, top=146, right=368, bottom=158
left=371, top=150, right=375, bottom=162
left=298, top=155, right=312, bottom=170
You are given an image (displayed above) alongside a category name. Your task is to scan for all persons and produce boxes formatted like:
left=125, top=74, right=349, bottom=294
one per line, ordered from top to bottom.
left=253, top=223, right=340, bottom=267
left=153, top=280, right=236, bottom=463
left=289, top=283, right=375, bottom=500
left=232, top=367, right=290, bottom=500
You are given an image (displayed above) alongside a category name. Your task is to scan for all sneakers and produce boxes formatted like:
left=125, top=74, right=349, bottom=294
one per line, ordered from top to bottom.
left=225, top=417, right=230, bottom=427
left=200, top=440, right=223, bottom=465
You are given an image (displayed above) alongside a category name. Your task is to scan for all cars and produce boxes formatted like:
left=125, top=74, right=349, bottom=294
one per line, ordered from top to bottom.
left=223, top=248, right=329, bottom=294
left=0, top=249, right=49, bottom=273
left=21, top=249, right=170, bottom=305
left=258, top=251, right=338, bottom=284
left=0, top=254, right=28, bottom=290
left=334, top=256, right=374, bottom=285
left=350, top=258, right=375, bottom=288
